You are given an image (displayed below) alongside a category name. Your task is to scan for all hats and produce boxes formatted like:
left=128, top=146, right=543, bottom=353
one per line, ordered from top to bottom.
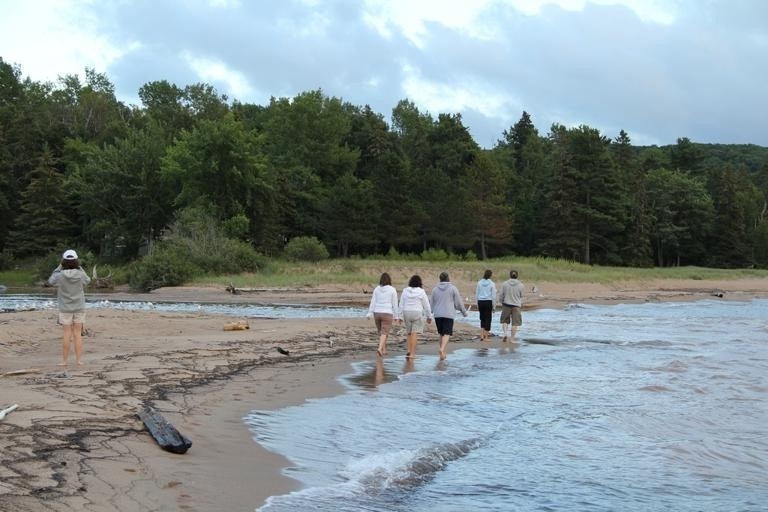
left=62, top=249, right=79, bottom=261
left=510, top=270, right=518, bottom=278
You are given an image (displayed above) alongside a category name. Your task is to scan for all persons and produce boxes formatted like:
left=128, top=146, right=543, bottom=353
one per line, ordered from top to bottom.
left=46, top=249, right=93, bottom=369
left=473, top=269, right=498, bottom=343
left=498, top=269, right=524, bottom=343
left=363, top=271, right=400, bottom=359
left=429, top=271, right=469, bottom=360
left=398, top=274, right=433, bottom=360
